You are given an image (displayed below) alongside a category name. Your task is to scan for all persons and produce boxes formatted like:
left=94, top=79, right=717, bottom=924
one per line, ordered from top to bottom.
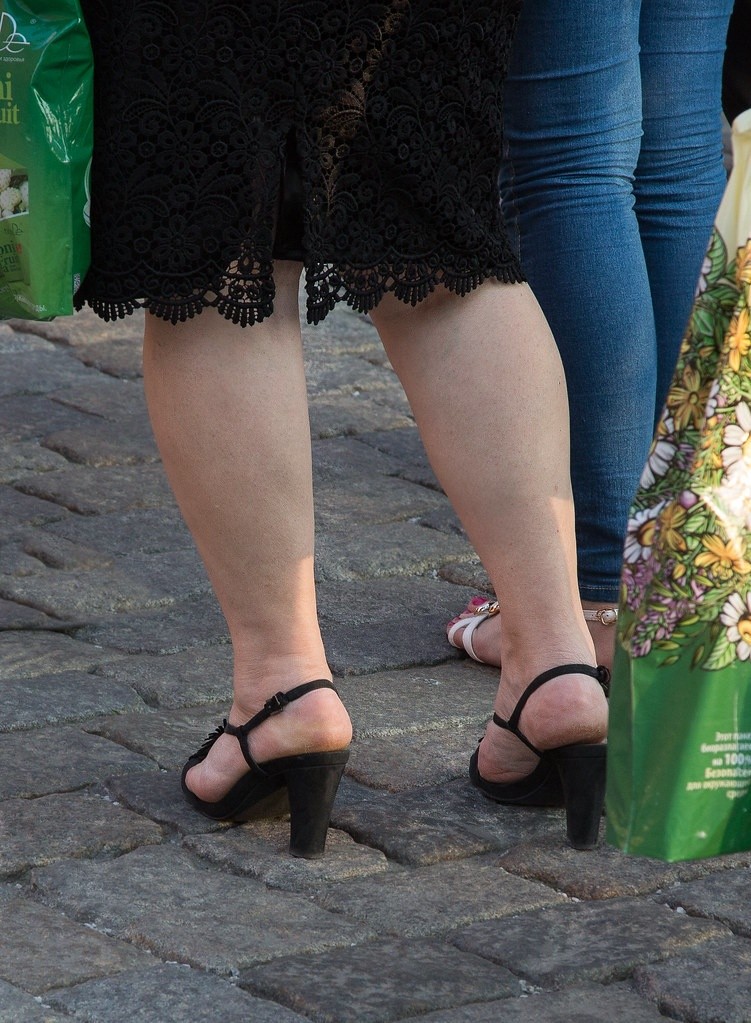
left=449, top=0, right=738, bottom=667
left=75, top=1, right=606, bottom=859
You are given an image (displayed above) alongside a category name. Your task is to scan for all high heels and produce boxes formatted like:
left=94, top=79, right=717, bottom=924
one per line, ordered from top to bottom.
left=469, top=664, right=611, bottom=851
left=181, top=679, right=350, bottom=859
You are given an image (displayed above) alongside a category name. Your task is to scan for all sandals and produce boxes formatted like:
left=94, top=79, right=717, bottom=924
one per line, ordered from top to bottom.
left=448, top=600, right=620, bottom=664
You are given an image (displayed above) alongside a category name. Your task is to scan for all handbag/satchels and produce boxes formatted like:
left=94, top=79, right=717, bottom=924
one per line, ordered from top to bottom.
left=0, top=0, right=93, bottom=323
left=605, top=103, right=751, bottom=865
